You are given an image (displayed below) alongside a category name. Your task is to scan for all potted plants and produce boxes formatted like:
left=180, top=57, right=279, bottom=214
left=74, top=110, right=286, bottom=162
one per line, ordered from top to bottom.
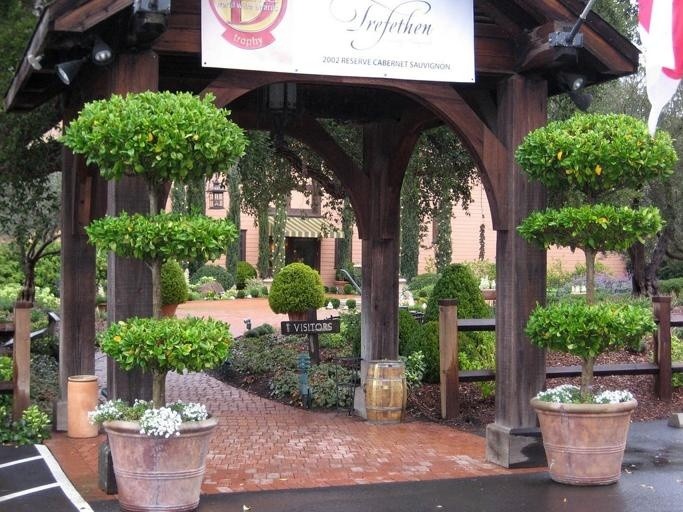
left=60, top=89, right=249, bottom=512
left=512, top=113, right=679, bottom=485
left=158, top=260, right=188, bottom=319
left=268, top=263, right=325, bottom=322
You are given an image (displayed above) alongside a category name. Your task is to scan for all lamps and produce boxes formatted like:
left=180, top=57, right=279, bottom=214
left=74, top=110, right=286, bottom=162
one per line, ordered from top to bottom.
left=208, top=180, right=224, bottom=211
